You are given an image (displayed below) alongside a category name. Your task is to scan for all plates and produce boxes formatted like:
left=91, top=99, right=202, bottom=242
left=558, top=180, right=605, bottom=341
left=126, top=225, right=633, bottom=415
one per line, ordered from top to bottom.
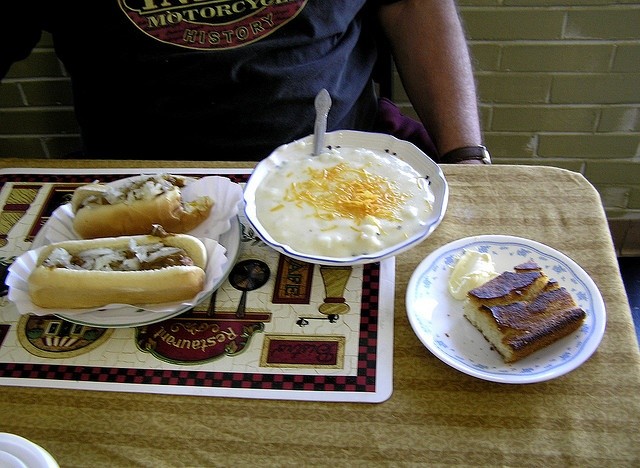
left=32, top=174, right=242, bottom=328
left=405, top=235, right=607, bottom=384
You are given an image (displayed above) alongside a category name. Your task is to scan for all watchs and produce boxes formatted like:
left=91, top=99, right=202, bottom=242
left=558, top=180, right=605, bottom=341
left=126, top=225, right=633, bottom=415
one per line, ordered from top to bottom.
left=438, top=146, right=492, bottom=164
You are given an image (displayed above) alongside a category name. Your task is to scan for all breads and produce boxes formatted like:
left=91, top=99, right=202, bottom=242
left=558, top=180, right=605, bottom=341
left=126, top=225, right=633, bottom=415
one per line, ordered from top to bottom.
left=71, top=174, right=215, bottom=238
left=27, top=222, right=206, bottom=308
left=464, top=258, right=586, bottom=362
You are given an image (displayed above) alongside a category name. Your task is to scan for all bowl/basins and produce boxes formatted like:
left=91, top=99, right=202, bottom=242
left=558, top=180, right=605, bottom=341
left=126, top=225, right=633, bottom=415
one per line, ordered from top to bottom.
left=242, top=130, right=450, bottom=265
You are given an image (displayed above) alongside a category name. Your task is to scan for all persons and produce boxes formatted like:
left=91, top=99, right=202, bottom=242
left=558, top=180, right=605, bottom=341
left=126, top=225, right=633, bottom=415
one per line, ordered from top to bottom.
left=0, top=0, right=492, bottom=165
